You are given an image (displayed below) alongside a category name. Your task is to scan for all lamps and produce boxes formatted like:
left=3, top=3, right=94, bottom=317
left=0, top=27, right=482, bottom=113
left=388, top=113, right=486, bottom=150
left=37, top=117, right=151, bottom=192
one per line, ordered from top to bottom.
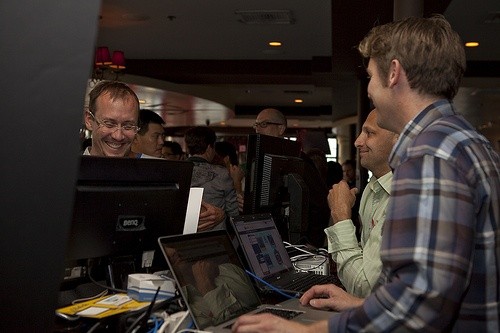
left=89, top=47, right=126, bottom=88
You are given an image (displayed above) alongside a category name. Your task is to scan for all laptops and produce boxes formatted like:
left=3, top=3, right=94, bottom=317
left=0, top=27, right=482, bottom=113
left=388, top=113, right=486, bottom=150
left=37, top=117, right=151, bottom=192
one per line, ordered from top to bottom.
left=228, top=211, right=345, bottom=297
left=158, top=229, right=340, bottom=333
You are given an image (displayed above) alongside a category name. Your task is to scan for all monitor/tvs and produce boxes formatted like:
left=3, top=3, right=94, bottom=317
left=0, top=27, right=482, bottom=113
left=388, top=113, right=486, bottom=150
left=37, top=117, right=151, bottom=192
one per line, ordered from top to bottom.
left=243, top=132, right=309, bottom=257
left=67, top=155, right=195, bottom=295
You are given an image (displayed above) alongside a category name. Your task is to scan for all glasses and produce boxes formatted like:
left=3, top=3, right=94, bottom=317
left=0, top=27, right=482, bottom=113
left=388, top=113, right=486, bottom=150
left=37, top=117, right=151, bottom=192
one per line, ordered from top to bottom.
left=88, top=110, right=141, bottom=137
left=252, top=121, right=284, bottom=128
left=161, top=151, right=174, bottom=159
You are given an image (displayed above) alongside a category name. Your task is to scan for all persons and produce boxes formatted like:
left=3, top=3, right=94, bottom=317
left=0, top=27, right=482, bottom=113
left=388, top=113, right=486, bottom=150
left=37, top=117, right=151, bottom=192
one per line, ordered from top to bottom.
left=232, top=14, right=500, bottom=333
left=82, top=81, right=242, bottom=233
left=162, top=240, right=258, bottom=328
